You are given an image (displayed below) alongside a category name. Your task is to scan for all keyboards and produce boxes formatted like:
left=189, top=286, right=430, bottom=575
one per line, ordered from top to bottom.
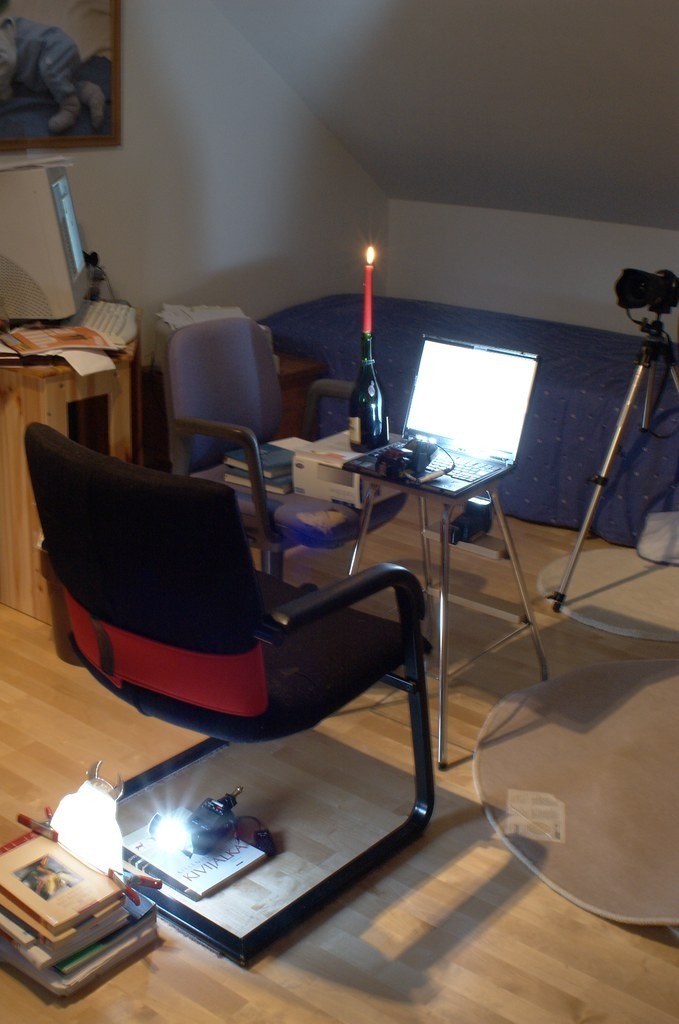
left=63, top=300, right=139, bottom=345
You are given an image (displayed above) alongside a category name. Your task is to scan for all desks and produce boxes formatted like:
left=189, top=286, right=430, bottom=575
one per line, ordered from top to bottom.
left=0, top=297, right=146, bottom=628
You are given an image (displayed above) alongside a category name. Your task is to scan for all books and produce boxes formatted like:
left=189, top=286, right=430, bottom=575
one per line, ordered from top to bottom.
left=224, top=434, right=314, bottom=477
left=223, top=468, right=294, bottom=495
left=119, top=806, right=267, bottom=901
left=0, top=828, right=161, bottom=998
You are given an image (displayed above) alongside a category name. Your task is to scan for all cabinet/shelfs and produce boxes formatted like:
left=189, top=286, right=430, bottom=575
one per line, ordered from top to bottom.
left=272, top=352, right=328, bottom=440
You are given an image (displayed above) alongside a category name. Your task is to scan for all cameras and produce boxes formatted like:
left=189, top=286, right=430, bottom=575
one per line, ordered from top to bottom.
left=614, top=267, right=679, bottom=314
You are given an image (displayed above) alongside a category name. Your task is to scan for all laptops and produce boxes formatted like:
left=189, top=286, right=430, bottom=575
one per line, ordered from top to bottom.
left=342, top=333, right=540, bottom=499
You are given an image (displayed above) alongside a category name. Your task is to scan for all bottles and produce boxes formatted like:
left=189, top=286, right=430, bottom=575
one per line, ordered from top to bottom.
left=350, top=332, right=390, bottom=453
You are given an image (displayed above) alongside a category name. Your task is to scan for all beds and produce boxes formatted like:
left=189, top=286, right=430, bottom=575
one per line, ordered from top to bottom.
left=258, top=293, right=678, bottom=549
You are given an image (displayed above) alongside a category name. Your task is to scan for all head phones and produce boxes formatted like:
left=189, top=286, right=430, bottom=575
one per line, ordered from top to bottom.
left=374, top=437, right=456, bottom=484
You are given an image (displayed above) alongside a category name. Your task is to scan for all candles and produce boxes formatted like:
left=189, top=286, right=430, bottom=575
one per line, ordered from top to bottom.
left=363, top=246, right=376, bottom=335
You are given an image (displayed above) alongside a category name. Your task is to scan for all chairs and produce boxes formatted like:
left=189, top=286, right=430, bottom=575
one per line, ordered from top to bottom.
left=169, top=316, right=408, bottom=581
left=24, top=421, right=434, bottom=970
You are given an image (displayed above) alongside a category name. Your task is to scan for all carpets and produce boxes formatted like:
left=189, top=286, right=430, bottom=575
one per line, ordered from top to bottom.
left=536, top=548, right=679, bottom=644
left=471, top=658, right=679, bottom=926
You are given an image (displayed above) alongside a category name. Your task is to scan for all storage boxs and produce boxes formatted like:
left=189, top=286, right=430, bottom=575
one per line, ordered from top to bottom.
left=293, top=428, right=402, bottom=509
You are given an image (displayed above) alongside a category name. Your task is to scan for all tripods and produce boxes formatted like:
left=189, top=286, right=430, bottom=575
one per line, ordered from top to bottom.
left=551, top=313, right=679, bottom=614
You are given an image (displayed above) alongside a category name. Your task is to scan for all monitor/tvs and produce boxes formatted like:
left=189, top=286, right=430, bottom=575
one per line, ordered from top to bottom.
left=0, top=165, right=91, bottom=324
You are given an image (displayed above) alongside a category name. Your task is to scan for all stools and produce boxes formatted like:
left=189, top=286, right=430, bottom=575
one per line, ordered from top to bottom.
left=346, top=468, right=549, bottom=771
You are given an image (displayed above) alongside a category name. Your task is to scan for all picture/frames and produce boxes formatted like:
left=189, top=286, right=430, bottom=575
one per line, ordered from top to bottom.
left=0, top=0, right=122, bottom=151
